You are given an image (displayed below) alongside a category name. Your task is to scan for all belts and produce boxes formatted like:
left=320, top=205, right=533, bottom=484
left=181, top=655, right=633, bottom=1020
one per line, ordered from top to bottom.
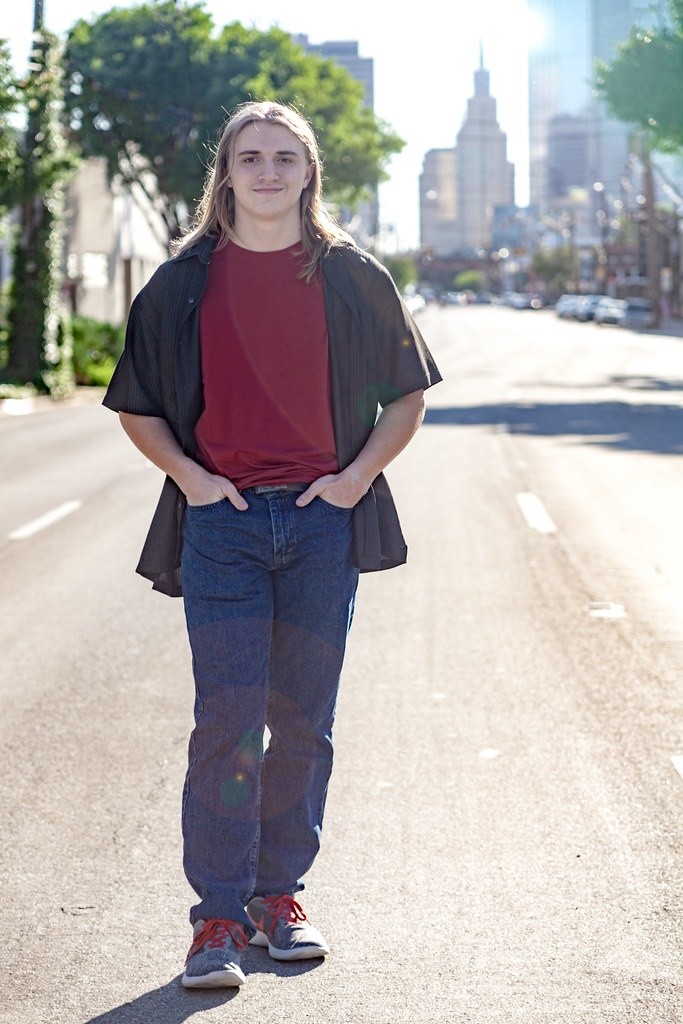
left=249, top=482, right=308, bottom=493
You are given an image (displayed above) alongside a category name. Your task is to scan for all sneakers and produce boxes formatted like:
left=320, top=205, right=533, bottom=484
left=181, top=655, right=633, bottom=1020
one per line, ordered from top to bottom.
left=247, top=892, right=330, bottom=959
left=181, top=918, right=249, bottom=987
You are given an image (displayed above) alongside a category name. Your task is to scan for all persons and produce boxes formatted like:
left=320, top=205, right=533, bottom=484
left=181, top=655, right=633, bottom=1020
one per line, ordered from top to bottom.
left=101, top=102, right=446, bottom=986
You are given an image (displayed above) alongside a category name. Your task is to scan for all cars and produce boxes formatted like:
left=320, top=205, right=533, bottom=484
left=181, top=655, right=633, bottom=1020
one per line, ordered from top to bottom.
left=554, top=294, right=655, bottom=327
